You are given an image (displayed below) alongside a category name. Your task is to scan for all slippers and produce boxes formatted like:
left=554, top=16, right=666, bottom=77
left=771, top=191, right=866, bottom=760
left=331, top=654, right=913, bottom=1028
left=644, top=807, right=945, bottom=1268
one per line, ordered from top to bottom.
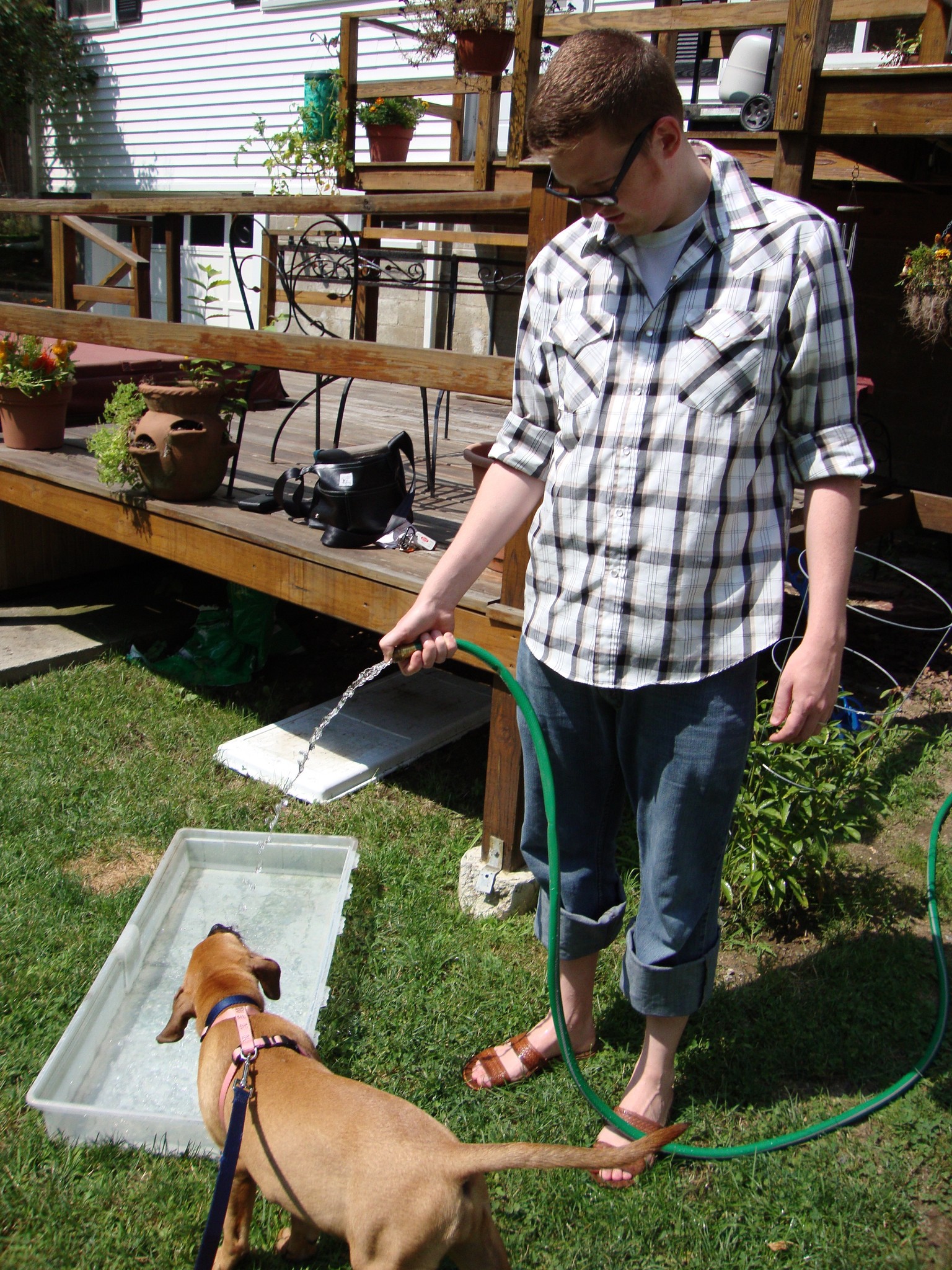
left=463, top=1028, right=595, bottom=1091
left=586, top=1105, right=665, bottom=1188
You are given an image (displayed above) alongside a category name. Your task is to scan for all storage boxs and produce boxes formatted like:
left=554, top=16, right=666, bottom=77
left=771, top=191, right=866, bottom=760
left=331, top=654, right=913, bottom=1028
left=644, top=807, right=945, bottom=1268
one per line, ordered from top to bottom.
left=26, top=824, right=360, bottom=1157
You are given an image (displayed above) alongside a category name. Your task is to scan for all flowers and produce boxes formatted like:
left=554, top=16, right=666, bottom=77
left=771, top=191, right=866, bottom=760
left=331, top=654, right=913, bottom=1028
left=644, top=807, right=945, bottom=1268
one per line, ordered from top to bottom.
left=353, top=95, right=430, bottom=127
left=894, top=234, right=952, bottom=296
left=0, top=291, right=81, bottom=398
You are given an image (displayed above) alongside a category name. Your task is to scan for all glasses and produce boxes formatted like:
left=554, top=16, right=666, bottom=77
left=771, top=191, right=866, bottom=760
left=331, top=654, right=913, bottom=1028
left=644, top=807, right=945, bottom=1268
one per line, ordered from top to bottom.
left=546, top=120, right=660, bottom=210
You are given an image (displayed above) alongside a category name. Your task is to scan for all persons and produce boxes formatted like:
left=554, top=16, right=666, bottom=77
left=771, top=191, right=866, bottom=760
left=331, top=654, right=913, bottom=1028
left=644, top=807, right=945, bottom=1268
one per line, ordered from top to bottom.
left=380, top=27, right=872, bottom=1188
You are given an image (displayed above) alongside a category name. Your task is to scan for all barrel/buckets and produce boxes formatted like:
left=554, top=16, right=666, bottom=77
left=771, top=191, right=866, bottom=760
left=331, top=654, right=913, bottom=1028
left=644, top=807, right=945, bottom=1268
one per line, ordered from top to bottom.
left=303, top=41, right=339, bottom=141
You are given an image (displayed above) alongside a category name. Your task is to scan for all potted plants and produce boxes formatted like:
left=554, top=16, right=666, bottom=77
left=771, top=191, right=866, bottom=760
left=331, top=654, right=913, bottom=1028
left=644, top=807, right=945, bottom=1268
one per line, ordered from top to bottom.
left=392, top=0, right=521, bottom=92
left=84, top=260, right=294, bottom=504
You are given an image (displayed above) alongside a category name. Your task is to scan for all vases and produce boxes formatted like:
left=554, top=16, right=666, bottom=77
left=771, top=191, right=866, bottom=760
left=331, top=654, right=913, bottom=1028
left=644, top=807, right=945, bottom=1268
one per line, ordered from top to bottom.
left=364, top=125, right=416, bottom=162
left=906, top=293, right=952, bottom=347
left=0, top=378, right=77, bottom=451
left=463, top=442, right=505, bottom=572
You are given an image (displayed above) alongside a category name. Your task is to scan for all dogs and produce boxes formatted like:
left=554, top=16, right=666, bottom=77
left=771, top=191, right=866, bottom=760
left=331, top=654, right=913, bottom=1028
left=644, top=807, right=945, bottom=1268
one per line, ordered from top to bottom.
left=153, top=921, right=695, bottom=1268
